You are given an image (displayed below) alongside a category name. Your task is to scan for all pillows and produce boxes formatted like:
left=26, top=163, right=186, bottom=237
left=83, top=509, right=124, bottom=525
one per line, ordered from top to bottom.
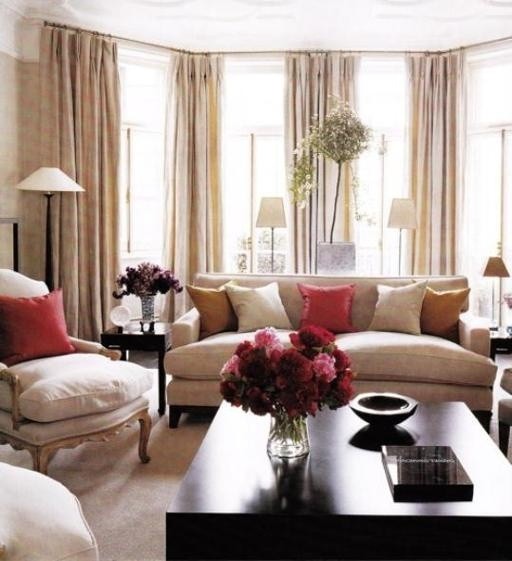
left=186, top=279, right=238, bottom=341
left=367, top=280, right=429, bottom=336
left=225, top=281, right=294, bottom=334
left=296, top=282, right=359, bottom=333
left=420, top=286, right=471, bottom=344
left=0, top=288, right=76, bottom=366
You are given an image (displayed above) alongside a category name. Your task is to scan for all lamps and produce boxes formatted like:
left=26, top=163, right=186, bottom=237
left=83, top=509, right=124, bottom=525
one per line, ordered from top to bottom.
left=16, top=167, right=87, bottom=293
left=256, top=197, right=287, bottom=273
left=387, top=198, right=417, bottom=276
left=483, top=257, right=510, bottom=330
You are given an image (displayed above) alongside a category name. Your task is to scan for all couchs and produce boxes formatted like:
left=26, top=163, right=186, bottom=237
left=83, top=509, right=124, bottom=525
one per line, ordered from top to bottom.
left=0, top=269, right=154, bottom=475
left=164, top=273, right=498, bottom=434
left=0, top=462, right=99, bottom=561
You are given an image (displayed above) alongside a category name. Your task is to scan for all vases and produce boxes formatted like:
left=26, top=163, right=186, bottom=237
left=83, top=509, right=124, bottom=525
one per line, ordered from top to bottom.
left=316, top=244, right=356, bottom=273
left=141, top=295, right=154, bottom=320
left=267, top=413, right=311, bottom=457
left=269, top=458, right=320, bottom=514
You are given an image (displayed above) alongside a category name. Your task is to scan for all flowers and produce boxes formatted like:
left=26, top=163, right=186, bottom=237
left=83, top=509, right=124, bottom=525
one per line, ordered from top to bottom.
left=289, top=92, right=373, bottom=243
left=112, top=262, right=183, bottom=299
left=220, top=323, right=357, bottom=418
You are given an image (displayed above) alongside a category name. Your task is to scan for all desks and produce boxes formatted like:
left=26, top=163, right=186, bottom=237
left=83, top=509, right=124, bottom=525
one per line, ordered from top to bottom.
left=100, top=321, right=171, bottom=415
left=490, top=338, right=512, bottom=362
left=166, top=400, right=511, bottom=561
